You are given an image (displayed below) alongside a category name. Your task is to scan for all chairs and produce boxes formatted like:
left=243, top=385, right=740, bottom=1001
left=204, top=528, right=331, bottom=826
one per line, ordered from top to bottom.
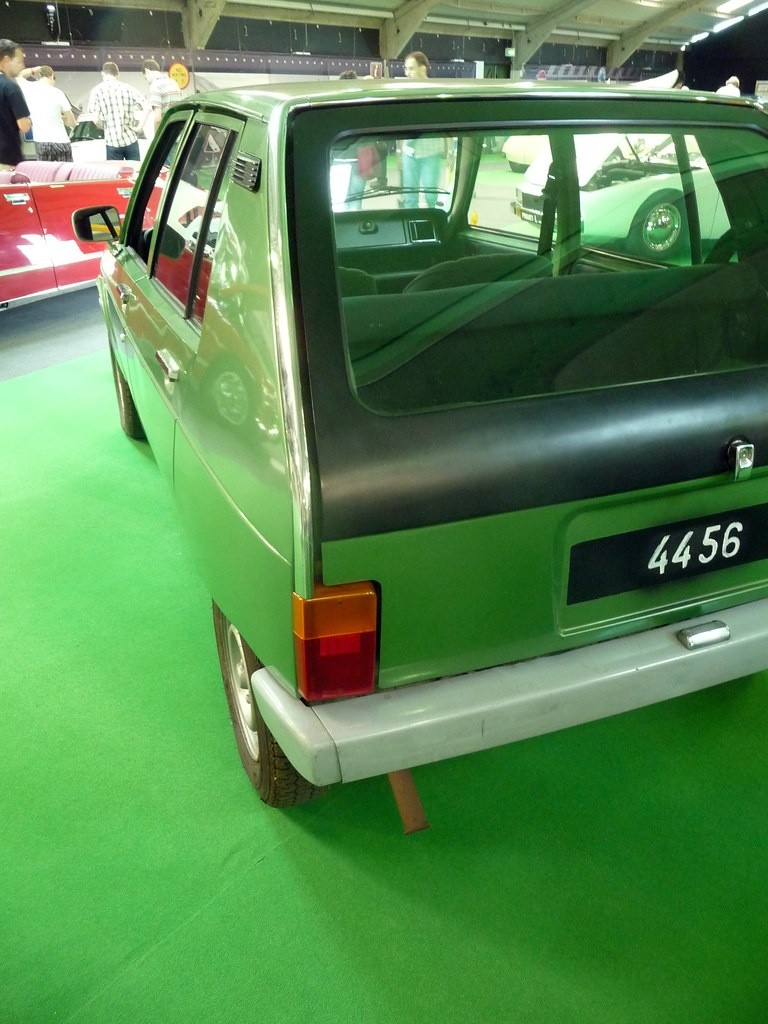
left=402, top=254, right=553, bottom=293
left=339, top=267, right=377, bottom=297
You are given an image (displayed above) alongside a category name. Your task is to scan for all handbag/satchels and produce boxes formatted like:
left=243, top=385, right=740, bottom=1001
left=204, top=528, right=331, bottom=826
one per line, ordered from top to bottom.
left=358, top=143, right=381, bottom=180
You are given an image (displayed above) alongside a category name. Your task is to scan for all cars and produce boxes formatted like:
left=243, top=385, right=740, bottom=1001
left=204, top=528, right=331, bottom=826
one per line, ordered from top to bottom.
left=502, top=69, right=740, bottom=175
left=70, top=78, right=768, bottom=810
left=511, top=87, right=768, bottom=262
left=71, top=108, right=155, bottom=162
left=0, top=161, right=225, bottom=314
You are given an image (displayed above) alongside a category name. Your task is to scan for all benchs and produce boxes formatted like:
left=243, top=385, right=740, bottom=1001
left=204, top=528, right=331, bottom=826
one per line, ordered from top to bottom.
left=14, top=161, right=143, bottom=183
left=343, top=263, right=768, bottom=412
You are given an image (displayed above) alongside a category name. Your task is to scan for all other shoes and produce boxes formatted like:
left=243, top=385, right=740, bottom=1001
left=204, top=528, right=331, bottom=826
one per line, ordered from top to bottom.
left=370, top=179, right=387, bottom=188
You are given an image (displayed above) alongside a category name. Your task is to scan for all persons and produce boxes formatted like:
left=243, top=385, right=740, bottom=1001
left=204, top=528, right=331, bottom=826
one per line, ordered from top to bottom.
left=0, top=38, right=187, bottom=168
left=338, top=49, right=458, bottom=218
